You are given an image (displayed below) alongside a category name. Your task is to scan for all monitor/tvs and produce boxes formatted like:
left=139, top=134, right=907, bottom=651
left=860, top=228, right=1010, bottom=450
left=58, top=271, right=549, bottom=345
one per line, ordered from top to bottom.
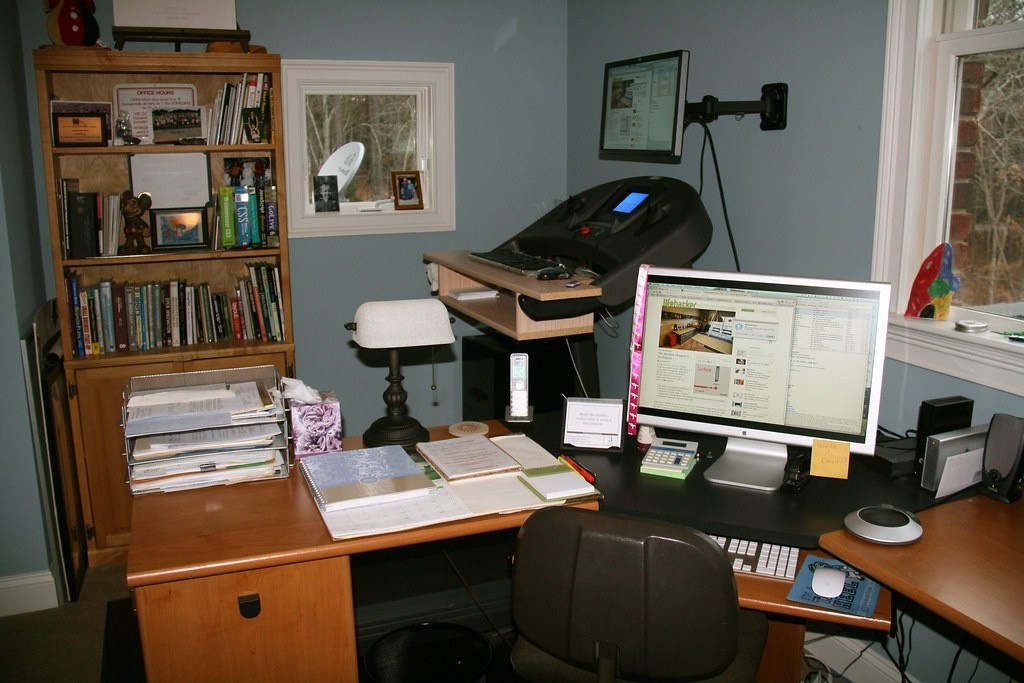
left=598, top=50, right=690, bottom=166
left=607, top=187, right=655, bottom=216
left=624, top=262, right=892, bottom=492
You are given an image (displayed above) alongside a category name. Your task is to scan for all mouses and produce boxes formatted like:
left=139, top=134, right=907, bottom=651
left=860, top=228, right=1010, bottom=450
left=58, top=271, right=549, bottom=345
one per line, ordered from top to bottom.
left=533, top=267, right=570, bottom=281
left=811, top=565, right=846, bottom=599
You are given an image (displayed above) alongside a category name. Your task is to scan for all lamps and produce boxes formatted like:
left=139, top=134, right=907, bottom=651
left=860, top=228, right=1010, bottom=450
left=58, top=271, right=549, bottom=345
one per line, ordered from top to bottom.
left=343, top=298, right=456, bottom=456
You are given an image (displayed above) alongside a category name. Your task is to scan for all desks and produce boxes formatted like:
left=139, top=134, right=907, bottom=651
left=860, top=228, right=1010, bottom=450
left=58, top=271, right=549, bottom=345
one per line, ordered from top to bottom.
left=125, top=423, right=1024, bottom=683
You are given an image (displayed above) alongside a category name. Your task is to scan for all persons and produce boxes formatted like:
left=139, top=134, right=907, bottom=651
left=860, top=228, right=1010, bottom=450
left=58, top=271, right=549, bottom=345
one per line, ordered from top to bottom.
left=102, top=109, right=108, bottom=125
left=248, top=111, right=258, bottom=130
left=155, top=113, right=197, bottom=128
left=251, top=124, right=260, bottom=136
left=252, top=130, right=260, bottom=143
left=251, top=159, right=266, bottom=185
left=229, top=161, right=244, bottom=186
left=400, top=176, right=415, bottom=200
left=316, top=183, right=339, bottom=212
left=696, top=318, right=710, bottom=332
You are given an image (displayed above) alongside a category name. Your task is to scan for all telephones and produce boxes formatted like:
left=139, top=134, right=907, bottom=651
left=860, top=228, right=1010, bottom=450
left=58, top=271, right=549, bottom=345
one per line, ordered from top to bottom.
left=505, top=352, right=534, bottom=423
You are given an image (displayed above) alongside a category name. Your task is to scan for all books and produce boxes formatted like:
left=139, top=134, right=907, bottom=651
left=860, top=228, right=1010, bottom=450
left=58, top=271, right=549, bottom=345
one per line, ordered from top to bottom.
left=207, top=72, right=275, bottom=145
left=56, top=177, right=121, bottom=260
left=202, top=184, right=279, bottom=252
left=64, top=271, right=285, bottom=356
left=447, top=288, right=498, bottom=300
left=415, top=435, right=522, bottom=484
left=299, top=446, right=438, bottom=514
left=640, top=459, right=696, bottom=480
left=516, top=466, right=600, bottom=499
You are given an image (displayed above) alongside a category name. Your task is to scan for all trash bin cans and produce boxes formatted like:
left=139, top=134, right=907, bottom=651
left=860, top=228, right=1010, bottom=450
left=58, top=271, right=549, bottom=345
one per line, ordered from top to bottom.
left=364, top=622, right=492, bottom=683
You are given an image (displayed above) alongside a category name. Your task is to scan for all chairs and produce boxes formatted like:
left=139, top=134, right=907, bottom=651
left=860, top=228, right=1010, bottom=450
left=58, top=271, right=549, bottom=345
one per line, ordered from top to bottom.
left=506, top=504, right=804, bottom=683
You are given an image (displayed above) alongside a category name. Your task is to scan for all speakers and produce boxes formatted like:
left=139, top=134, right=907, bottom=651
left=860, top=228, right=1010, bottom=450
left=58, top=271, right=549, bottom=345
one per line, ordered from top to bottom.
left=975, top=413, right=1024, bottom=505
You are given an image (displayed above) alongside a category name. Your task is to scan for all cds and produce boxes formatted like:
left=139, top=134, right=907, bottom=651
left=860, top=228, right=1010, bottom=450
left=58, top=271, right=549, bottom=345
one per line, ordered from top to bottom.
left=954, top=320, right=987, bottom=334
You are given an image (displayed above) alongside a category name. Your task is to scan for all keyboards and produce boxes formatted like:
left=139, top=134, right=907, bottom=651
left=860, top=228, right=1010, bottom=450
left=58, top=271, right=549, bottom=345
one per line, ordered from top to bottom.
left=467, top=250, right=562, bottom=274
left=704, top=534, right=800, bottom=583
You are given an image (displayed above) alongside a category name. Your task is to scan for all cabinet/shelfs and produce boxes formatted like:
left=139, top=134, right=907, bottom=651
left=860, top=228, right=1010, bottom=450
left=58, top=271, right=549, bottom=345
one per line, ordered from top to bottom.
left=32, top=45, right=297, bottom=567
left=423, top=253, right=602, bottom=340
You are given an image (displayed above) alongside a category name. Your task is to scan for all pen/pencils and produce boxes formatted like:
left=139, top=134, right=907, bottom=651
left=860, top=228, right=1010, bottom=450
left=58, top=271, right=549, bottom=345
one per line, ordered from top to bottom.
left=558, top=454, right=598, bottom=483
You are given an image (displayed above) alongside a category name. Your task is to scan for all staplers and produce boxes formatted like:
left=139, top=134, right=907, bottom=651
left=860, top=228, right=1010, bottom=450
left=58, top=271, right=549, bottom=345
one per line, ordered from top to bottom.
left=783, top=450, right=811, bottom=490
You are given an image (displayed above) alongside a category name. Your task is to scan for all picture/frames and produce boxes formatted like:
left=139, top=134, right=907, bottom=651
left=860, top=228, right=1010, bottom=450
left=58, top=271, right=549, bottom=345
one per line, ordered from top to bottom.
left=390, top=171, right=425, bottom=210
left=148, top=207, right=211, bottom=251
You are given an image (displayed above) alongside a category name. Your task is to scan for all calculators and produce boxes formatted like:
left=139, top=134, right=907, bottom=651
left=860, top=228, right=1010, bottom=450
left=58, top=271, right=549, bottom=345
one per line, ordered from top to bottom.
left=641, top=437, right=699, bottom=470
